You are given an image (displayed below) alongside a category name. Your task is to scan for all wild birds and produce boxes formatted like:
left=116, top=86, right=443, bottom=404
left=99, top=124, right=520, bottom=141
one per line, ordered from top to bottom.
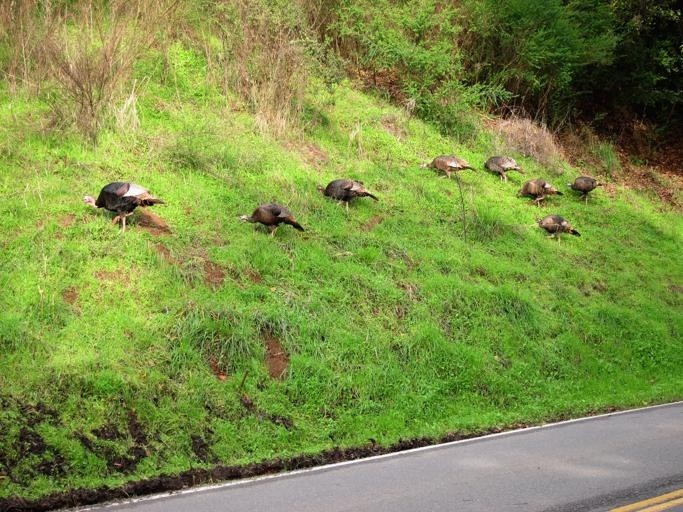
left=427, top=156, right=476, bottom=179
left=485, top=156, right=524, bottom=181
left=566, top=177, right=604, bottom=201
left=315, top=179, right=380, bottom=213
left=515, top=179, right=564, bottom=209
left=83, top=182, right=165, bottom=233
left=239, top=204, right=305, bottom=240
left=534, top=215, right=580, bottom=243
